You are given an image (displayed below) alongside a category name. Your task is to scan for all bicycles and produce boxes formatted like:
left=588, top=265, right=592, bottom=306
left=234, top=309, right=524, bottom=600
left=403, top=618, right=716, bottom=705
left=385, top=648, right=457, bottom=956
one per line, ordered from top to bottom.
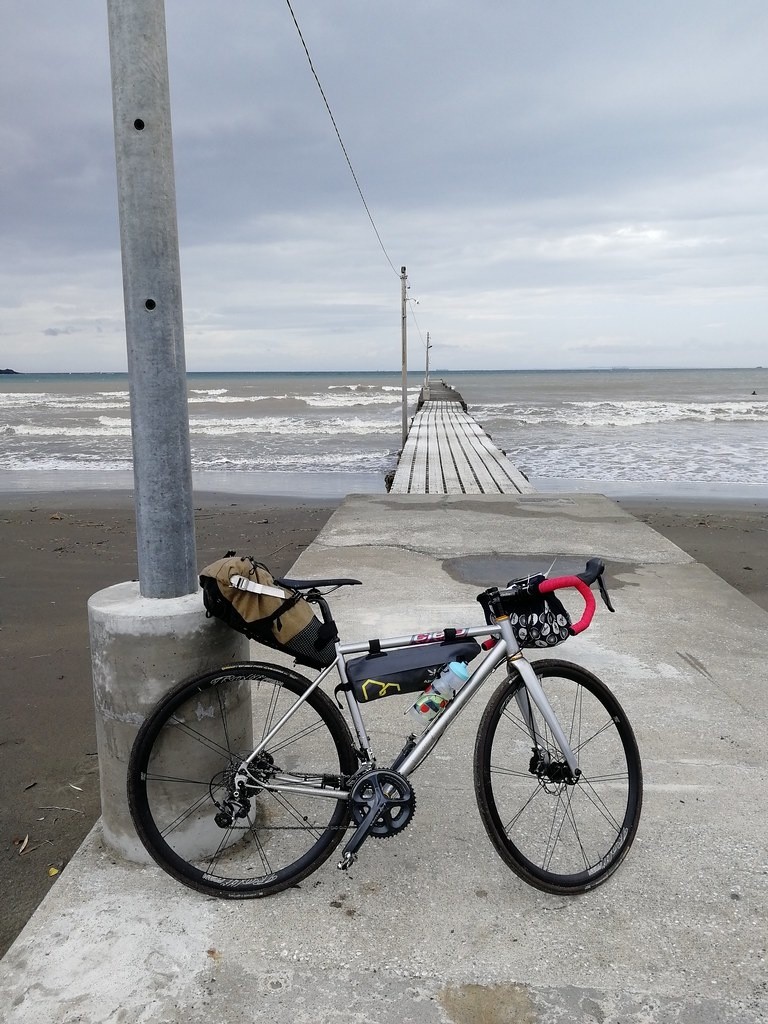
left=128, top=558, right=642, bottom=897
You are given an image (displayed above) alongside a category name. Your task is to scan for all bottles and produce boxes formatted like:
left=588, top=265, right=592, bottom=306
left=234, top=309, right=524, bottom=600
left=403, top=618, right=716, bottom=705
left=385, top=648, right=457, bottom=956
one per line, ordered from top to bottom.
left=411, top=660, right=469, bottom=724
